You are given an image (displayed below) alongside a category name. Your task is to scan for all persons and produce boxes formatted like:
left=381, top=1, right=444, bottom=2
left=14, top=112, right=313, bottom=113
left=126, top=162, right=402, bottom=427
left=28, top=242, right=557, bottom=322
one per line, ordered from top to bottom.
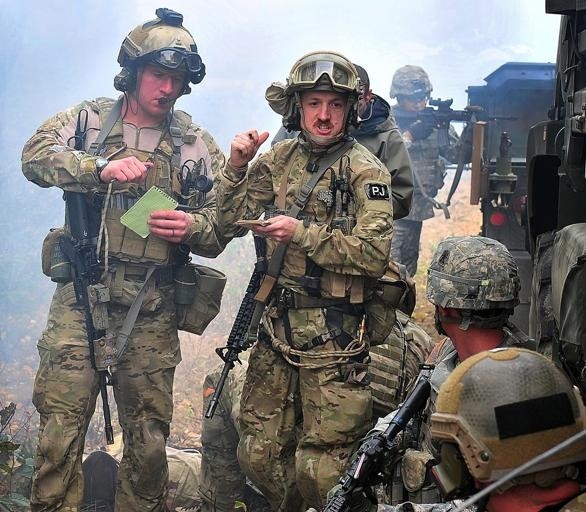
left=18, top=6, right=227, bottom=511
left=217, top=49, right=398, bottom=511
left=267, top=62, right=416, bottom=224
left=388, top=63, right=490, bottom=284
left=327, top=233, right=536, bottom=510
left=176, top=260, right=436, bottom=512
left=425, top=344, right=586, bottom=512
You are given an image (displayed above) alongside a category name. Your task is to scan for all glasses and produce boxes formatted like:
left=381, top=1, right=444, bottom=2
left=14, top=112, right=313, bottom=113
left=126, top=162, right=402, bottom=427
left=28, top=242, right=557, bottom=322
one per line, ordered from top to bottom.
left=133, top=47, right=203, bottom=73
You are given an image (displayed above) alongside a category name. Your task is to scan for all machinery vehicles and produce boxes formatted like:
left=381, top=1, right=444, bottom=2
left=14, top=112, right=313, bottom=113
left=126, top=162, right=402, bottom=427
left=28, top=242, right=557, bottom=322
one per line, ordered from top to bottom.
left=458, top=0, right=586, bottom=365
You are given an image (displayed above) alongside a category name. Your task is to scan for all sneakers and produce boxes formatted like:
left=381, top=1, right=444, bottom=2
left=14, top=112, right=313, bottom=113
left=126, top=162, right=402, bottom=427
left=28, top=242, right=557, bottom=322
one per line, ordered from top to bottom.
left=174, top=499, right=222, bottom=512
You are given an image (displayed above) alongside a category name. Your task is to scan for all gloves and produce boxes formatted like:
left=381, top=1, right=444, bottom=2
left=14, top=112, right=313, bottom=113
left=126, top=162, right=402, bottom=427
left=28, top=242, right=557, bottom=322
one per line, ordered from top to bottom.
left=465, top=106, right=487, bottom=127
left=408, top=118, right=435, bottom=142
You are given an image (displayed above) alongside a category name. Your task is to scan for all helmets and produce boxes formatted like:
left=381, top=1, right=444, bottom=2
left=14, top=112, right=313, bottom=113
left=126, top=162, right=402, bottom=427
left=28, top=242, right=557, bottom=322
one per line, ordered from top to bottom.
left=117, top=5, right=199, bottom=76
left=285, top=50, right=360, bottom=99
left=390, top=65, right=433, bottom=98
left=426, top=235, right=522, bottom=308
left=385, top=259, right=416, bottom=317
left=422, top=344, right=585, bottom=488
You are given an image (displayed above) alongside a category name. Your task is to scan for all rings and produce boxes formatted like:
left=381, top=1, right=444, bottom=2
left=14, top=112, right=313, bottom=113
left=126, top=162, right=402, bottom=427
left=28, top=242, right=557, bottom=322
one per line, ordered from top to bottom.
left=171, top=228, right=177, bottom=238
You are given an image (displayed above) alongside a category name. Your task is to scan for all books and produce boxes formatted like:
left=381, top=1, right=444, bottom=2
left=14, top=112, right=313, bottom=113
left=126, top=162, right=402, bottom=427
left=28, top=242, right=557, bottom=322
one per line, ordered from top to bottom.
left=115, top=184, right=180, bottom=240
left=229, top=215, right=268, bottom=228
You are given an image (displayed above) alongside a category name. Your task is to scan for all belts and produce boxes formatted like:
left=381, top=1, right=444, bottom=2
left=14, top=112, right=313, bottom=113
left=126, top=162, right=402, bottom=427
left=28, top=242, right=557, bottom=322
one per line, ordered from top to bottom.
left=277, top=291, right=345, bottom=310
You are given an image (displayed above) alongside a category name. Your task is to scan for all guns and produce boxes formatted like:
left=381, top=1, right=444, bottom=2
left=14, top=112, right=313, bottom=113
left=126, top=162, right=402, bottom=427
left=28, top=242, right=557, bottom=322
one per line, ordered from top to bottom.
left=391, top=98, right=518, bottom=149
left=63, top=136, right=114, bottom=446
left=204, top=198, right=277, bottom=419
left=318, top=379, right=431, bottom=512
